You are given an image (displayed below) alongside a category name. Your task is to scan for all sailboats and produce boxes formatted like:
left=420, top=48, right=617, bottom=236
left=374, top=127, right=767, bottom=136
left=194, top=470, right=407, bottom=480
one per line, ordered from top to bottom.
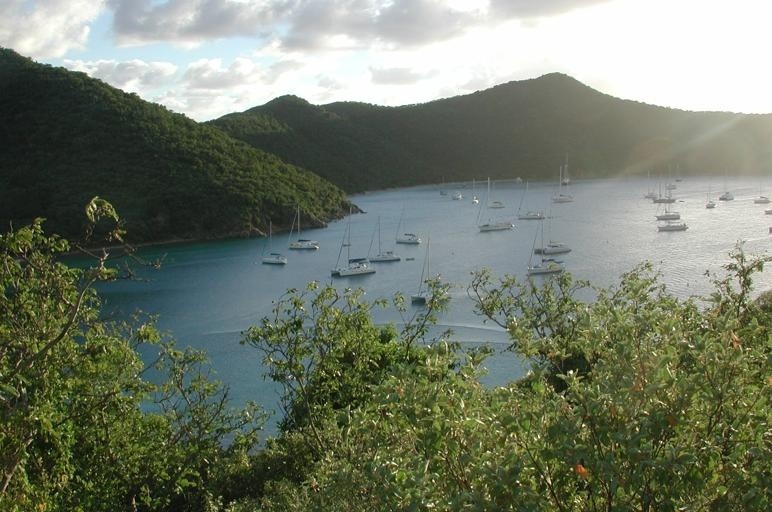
left=436, top=154, right=576, bottom=233
left=640, top=163, right=772, bottom=236
left=287, top=198, right=320, bottom=250
left=333, top=205, right=378, bottom=279
left=533, top=211, right=573, bottom=256
left=395, top=213, right=423, bottom=245
left=365, top=215, right=402, bottom=263
left=524, top=218, right=566, bottom=276
left=260, top=220, right=289, bottom=265
left=410, top=237, right=450, bottom=305
